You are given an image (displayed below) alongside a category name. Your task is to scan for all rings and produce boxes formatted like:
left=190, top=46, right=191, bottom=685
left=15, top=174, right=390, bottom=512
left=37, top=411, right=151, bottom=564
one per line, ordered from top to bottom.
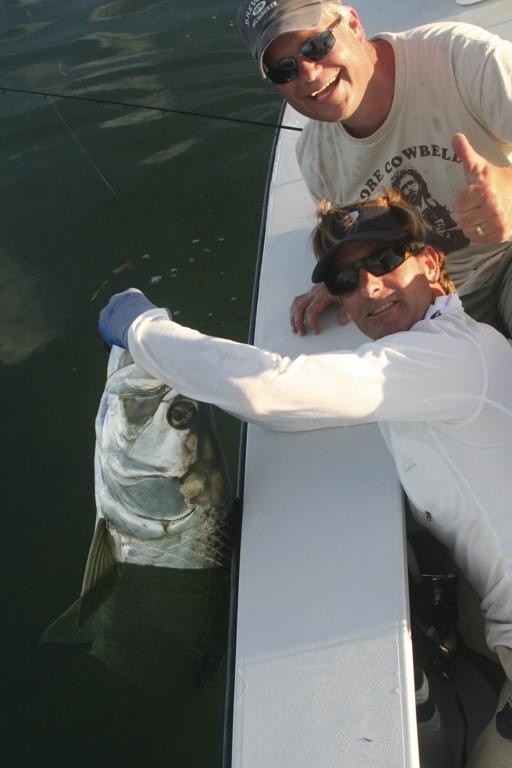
left=476, top=225, right=488, bottom=241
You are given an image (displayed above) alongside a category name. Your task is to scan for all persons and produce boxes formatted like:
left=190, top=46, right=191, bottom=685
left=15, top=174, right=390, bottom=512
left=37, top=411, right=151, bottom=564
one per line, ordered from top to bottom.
left=232, top=4, right=511, bottom=338
left=100, top=191, right=512, bottom=768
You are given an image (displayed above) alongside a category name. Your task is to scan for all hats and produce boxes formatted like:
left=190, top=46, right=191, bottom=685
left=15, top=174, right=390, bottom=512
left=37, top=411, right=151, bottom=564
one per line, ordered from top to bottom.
left=238, top=0, right=320, bottom=81
left=312, top=206, right=412, bottom=283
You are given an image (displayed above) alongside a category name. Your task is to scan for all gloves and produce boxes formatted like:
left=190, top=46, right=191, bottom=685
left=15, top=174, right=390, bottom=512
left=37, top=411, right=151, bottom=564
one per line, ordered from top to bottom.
left=98, top=288, right=159, bottom=349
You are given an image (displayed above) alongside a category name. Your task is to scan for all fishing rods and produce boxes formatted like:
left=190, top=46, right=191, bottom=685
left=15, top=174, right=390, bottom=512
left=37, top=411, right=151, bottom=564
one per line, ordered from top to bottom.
left=1, top=87, right=301, bottom=132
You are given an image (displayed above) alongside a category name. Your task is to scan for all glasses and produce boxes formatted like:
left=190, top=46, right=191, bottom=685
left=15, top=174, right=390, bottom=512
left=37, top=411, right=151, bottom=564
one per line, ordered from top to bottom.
left=267, top=16, right=342, bottom=85
left=322, top=237, right=424, bottom=296
